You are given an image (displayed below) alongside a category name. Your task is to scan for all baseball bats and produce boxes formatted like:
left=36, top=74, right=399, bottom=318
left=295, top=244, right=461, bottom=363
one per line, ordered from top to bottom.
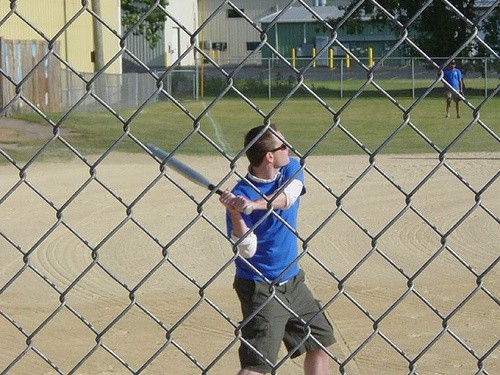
left=143, top=141, right=253, bottom=215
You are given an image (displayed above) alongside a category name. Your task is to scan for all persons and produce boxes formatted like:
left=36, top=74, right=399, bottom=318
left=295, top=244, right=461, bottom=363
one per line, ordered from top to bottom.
left=444, top=57, right=464, bottom=119
left=219, top=122, right=336, bottom=375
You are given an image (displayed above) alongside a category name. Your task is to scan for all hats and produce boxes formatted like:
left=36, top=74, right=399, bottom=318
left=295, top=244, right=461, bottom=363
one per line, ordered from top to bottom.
left=450, top=59, right=455, bottom=64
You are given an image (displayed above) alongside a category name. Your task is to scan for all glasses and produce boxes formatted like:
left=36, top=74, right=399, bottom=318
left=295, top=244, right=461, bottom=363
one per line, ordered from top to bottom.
left=264, top=143, right=287, bottom=156
